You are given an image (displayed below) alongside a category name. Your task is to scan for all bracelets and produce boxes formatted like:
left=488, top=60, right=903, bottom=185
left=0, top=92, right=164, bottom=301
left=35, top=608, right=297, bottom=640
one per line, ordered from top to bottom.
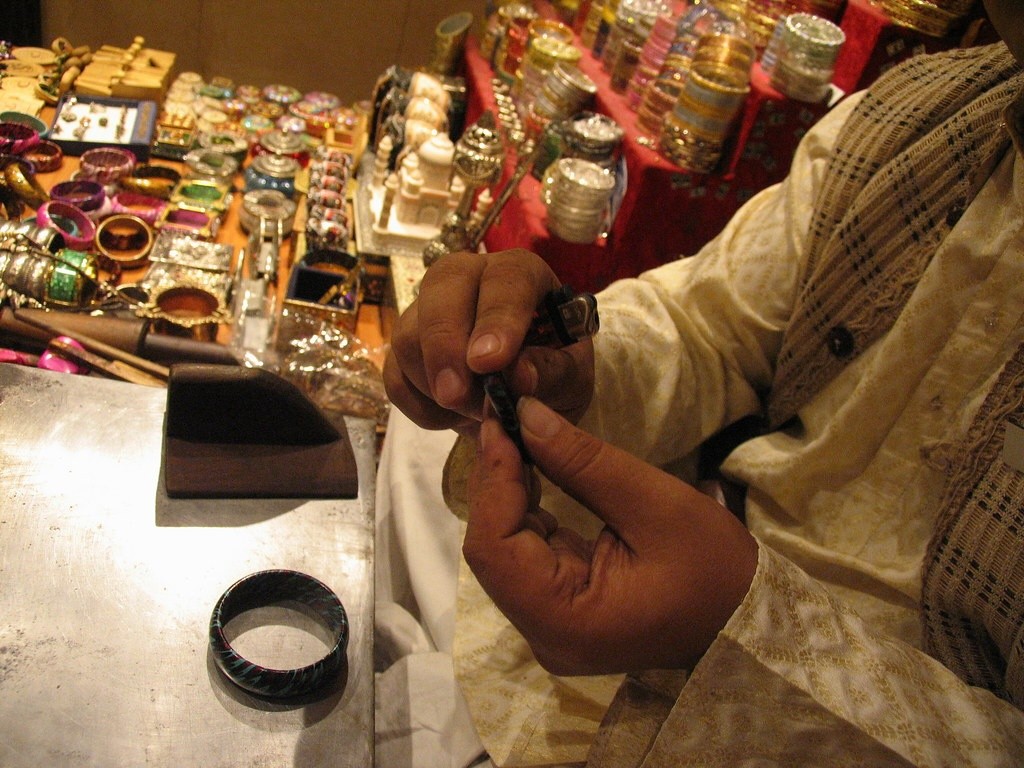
left=0, top=112, right=181, bottom=268
left=210, top=569, right=349, bottom=698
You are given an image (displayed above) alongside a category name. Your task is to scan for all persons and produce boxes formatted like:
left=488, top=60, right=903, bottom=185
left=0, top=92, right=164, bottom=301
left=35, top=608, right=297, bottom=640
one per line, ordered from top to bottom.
left=382, top=0, right=1023, bottom=768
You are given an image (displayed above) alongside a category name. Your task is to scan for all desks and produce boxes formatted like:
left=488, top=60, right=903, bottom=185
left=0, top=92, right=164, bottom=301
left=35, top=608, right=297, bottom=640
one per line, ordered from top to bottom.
left=0, top=361, right=376, bottom=768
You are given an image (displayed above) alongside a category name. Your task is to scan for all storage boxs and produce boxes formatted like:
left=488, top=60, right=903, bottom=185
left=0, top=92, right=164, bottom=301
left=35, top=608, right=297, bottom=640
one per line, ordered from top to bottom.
left=276, top=264, right=360, bottom=353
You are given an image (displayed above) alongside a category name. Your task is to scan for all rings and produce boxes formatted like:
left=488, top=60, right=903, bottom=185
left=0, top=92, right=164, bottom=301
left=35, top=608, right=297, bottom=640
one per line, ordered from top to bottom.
left=52, top=95, right=127, bottom=141
